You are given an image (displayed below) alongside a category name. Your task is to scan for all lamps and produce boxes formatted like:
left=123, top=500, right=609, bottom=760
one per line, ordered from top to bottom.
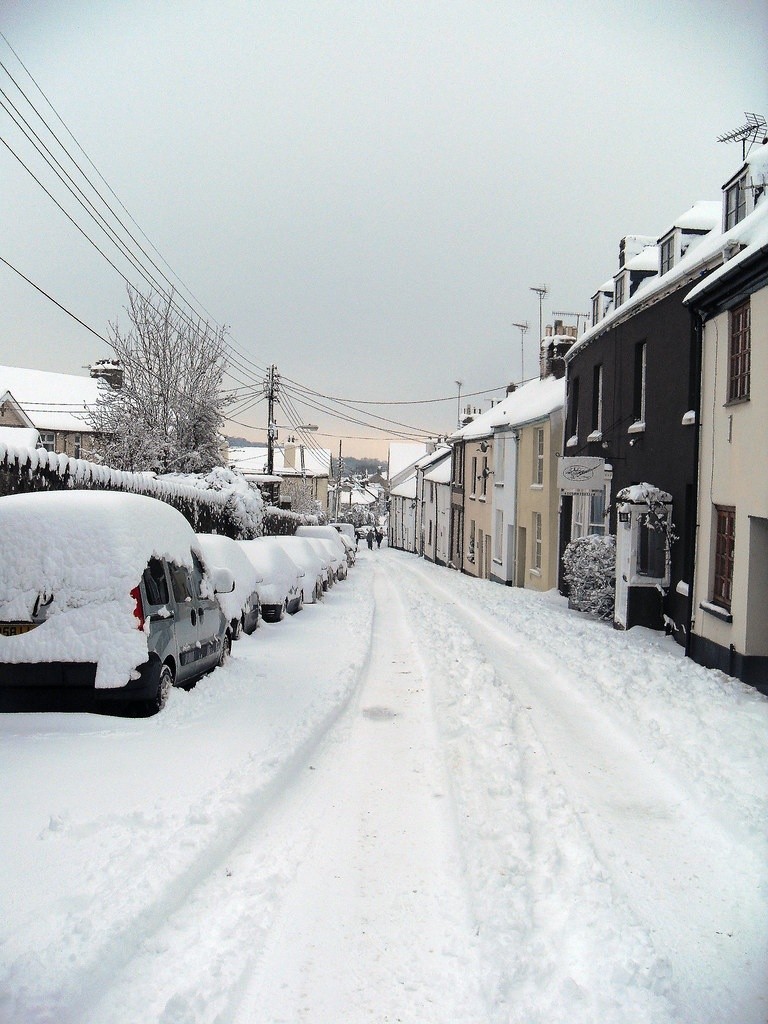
left=681, top=410, right=695, bottom=426
left=628, top=421, right=646, bottom=433
left=586, top=430, right=603, bottom=443
left=566, top=436, right=578, bottom=448
left=629, top=439, right=635, bottom=446
left=602, top=441, right=610, bottom=449
left=480, top=442, right=492, bottom=454
left=482, top=469, right=494, bottom=479
left=409, top=503, right=416, bottom=509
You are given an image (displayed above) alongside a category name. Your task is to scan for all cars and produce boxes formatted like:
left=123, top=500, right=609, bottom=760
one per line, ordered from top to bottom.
left=192, top=523, right=380, bottom=642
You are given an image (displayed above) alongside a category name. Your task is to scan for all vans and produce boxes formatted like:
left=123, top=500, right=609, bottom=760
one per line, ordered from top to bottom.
left=0, top=489, right=236, bottom=719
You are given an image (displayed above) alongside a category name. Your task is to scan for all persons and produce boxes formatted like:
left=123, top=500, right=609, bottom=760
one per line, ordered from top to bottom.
left=379, top=527, right=383, bottom=534
left=367, top=529, right=374, bottom=550
left=355, top=531, right=360, bottom=539
left=376, top=533, right=383, bottom=547
